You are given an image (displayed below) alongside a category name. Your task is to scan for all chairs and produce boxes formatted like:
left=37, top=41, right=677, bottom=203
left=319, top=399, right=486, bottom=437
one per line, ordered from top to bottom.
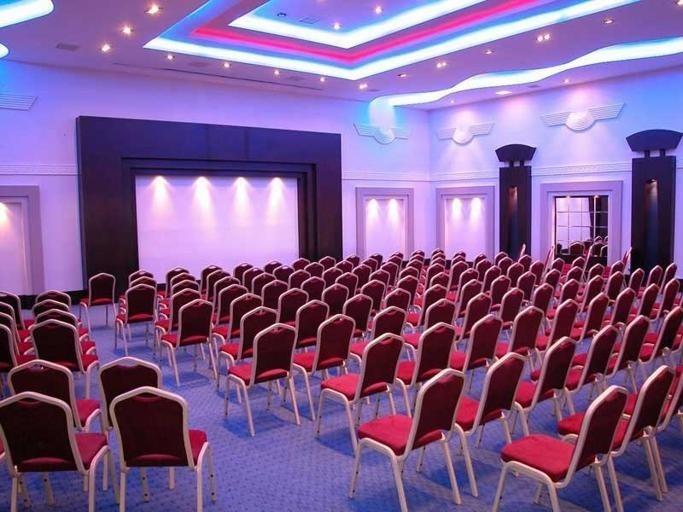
left=0, top=244, right=682, bottom=511
